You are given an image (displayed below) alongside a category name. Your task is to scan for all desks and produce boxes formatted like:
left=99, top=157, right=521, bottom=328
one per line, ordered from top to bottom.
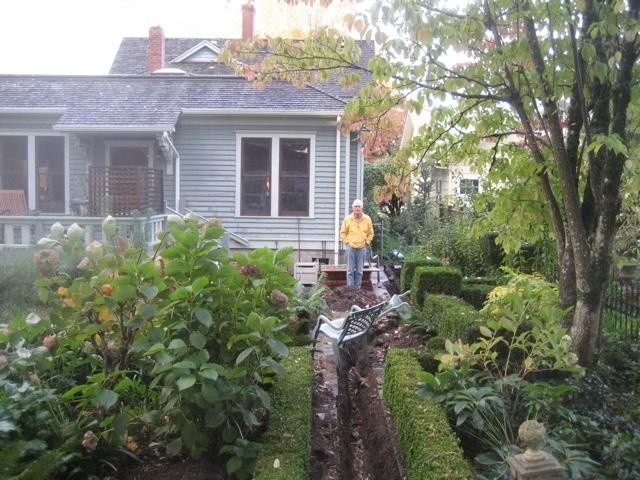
left=321, top=263, right=381, bottom=290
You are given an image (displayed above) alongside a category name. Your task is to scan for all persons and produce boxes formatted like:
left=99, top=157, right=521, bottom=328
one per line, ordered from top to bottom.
left=339, top=198, right=374, bottom=290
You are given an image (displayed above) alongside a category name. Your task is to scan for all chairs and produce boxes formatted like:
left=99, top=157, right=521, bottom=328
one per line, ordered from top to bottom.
left=0, top=189, right=40, bottom=231
left=310, top=302, right=385, bottom=377
